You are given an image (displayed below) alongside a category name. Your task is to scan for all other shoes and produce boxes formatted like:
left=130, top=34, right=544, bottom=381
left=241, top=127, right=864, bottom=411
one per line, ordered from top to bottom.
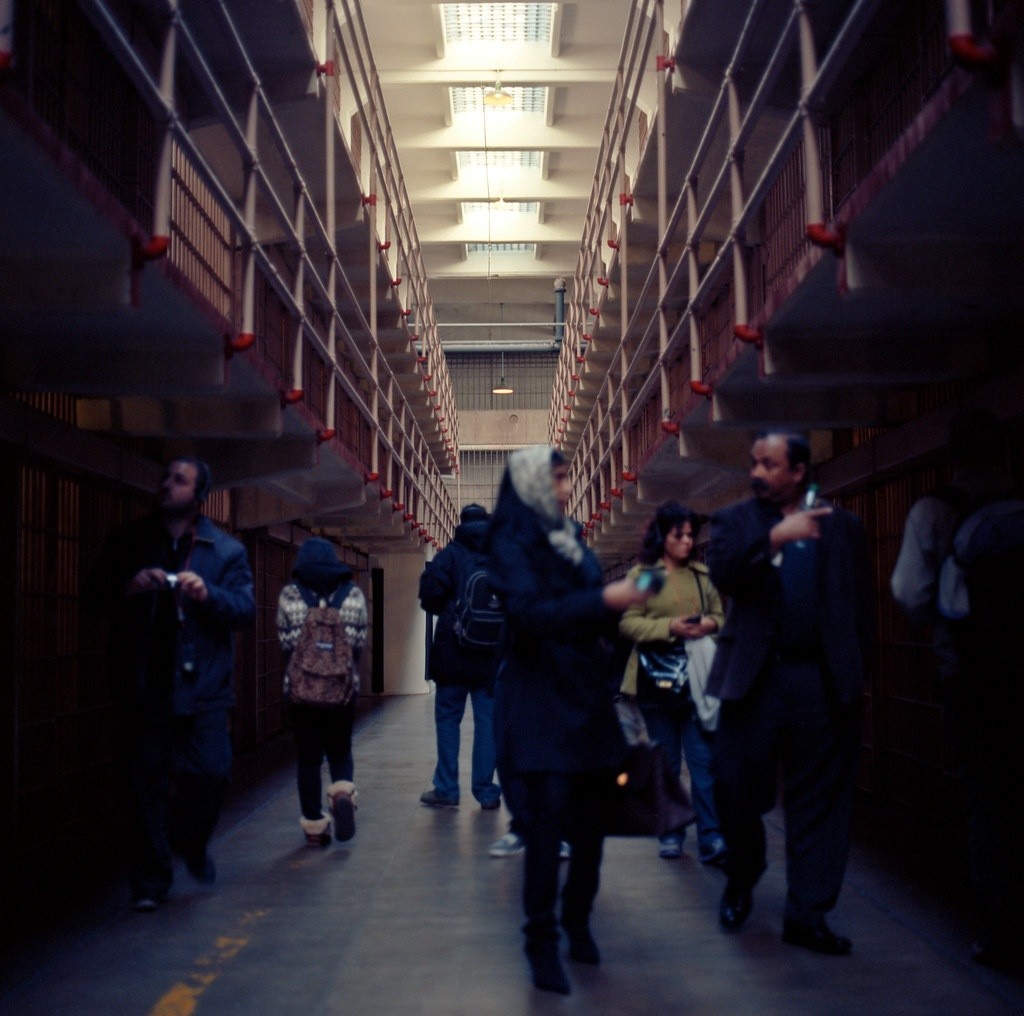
left=327, top=781, right=358, bottom=843
left=420, top=788, right=457, bottom=805
left=483, top=796, right=503, bottom=812
left=299, top=812, right=330, bottom=851
left=660, top=836, right=681, bottom=859
left=183, top=838, right=217, bottom=888
left=697, top=839, right=726, bottom=863
left=136, top=887, right=161, bottom=914
left=562, top=915, right=600, bottom=967
left=524, top=937, right=570, bottom=995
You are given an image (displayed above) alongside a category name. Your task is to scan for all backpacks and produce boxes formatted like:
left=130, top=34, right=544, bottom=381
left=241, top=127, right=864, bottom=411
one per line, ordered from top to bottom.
left=449, top=537, right=506, bottom=649
left=284, top=580, right=357, bottom=706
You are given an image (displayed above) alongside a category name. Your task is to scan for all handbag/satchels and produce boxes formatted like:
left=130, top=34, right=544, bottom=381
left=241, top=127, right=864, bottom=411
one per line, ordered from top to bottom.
left=634, top=638, right=696, bottom=714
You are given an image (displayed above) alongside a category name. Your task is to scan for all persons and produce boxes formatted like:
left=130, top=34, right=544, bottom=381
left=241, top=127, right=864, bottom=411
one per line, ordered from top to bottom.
left=891, top=408, right=1024, bottom=978
left=707, top=431, right=870, bottom=959
left=488, top=445, right=662, bottom=996
left=103, top=457, right=257, bottom=913
left=622, top=498, right=732, bottom=864
left=419, top=502, right=510, bottom=810
left=273, top=536, right=369, bottom=849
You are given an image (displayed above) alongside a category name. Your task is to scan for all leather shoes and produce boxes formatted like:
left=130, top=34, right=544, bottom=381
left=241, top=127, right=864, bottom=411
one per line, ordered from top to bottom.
left=720, top=878, right=758, bottom=929
left=783, top=919, right=835, bottom=953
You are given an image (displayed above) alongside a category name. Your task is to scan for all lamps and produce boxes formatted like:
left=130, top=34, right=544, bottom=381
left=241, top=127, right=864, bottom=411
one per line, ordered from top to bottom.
left=484, top=69, right=513, bottom=107
left=491, top=351, right=515, bottom=393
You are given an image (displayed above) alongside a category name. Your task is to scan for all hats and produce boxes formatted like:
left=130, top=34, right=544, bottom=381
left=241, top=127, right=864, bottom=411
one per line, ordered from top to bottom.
left=290, top=541, right=353, bottom=580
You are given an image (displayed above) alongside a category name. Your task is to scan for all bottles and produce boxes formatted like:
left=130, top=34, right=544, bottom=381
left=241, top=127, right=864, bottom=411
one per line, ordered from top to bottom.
left=780, top=483, right=821, bottom=592
